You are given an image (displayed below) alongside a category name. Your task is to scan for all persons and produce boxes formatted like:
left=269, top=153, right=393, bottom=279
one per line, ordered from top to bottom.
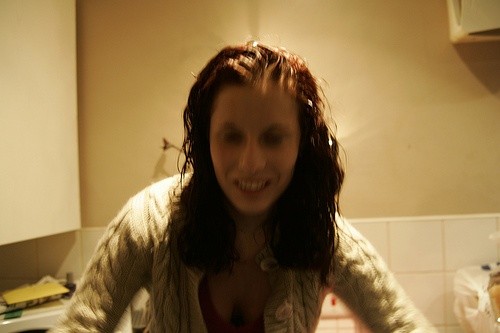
left=46, top=41, right=438, bottom=333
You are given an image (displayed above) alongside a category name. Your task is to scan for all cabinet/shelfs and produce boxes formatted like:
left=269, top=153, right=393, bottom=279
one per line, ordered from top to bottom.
left=0, top=0, right=82, bottom=333
